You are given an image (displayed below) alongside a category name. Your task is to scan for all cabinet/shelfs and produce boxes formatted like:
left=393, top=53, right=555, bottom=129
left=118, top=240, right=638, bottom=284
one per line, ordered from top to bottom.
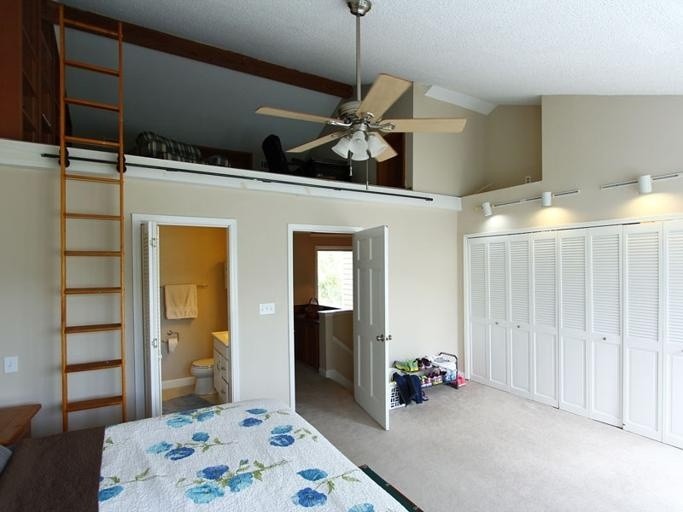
left=466, top=216, right=683, bottom=452
left=211, top=329, right=228, bottom=404
left=387, top=352, right=459, bottom=409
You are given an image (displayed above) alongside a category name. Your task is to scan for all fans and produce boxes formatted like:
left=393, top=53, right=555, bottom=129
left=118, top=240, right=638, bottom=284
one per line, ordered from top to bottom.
left=254, top=0, right=466, bottom=163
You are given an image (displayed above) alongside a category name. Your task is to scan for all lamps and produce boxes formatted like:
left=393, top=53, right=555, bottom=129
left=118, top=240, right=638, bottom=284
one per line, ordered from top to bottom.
left=331, top=0, right=386, bottom=162
left=637, top=173, right=652, bottom=194
left=540, top=190, right=551, bottom=206
left=480, top=201, right=491, bottom=217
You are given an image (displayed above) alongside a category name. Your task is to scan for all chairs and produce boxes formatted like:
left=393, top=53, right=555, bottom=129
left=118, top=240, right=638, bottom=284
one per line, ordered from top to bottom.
left=262, top=135, right=351, bottom=182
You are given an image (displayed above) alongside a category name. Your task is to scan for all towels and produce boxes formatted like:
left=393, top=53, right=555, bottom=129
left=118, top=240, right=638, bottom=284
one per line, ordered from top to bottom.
left=164, top=283, right=197, bottom=320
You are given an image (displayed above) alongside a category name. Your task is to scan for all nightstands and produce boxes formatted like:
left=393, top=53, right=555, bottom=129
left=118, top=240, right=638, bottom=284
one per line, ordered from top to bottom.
left=0, top=405, right=41, bottom=447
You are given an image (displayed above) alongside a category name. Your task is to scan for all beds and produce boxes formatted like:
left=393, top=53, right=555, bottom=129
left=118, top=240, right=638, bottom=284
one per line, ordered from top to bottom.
left=0, top=398, right=409, bottom=512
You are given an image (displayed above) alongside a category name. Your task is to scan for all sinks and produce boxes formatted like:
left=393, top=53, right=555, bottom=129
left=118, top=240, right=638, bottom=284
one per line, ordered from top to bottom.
left=213, top=331, right=229, bottom=346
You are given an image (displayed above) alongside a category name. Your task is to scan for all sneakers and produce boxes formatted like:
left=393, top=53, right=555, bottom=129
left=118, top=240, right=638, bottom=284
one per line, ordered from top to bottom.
left=395, top=357, right=433, bottom=371
left=418, top=371, right=456, bottom=387
left=452, top=375, right=466, bottom=386
left=421, top=392, right=427, bottom=401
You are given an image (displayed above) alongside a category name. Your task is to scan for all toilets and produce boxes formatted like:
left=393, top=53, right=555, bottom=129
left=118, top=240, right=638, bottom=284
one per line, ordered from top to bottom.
left=190, top=358, right=216, bottom=396
